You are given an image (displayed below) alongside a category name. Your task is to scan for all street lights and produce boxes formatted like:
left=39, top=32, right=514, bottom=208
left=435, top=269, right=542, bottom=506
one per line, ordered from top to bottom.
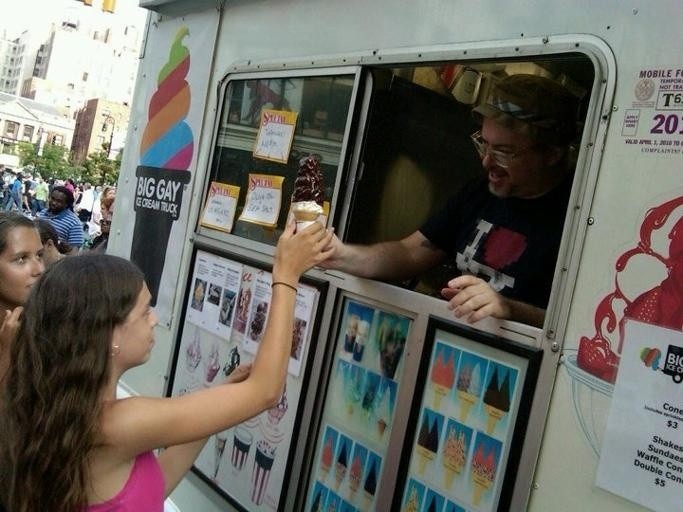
left=99, top=111, right=116, bottom=185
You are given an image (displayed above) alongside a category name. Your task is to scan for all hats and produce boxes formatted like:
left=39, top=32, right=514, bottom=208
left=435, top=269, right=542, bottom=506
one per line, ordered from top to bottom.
left=471, top=73, right=579, bottom=147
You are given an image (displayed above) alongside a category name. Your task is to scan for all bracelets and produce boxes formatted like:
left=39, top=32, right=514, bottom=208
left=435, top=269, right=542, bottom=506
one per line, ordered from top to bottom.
left=271, top=281, right=298, bottom=292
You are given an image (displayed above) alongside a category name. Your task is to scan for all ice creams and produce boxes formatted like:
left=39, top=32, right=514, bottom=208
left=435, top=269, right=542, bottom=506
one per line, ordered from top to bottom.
left=129, top=25, right=194, bottom=308
left=291, top=156, right=324, bottom=222
left=576, top=195, right=683, bottom=385
left=415, top=348, right=511, bottom=508
left=376, top=386, right=391, bottom=437
left=319, top=433, right=376, bottom=512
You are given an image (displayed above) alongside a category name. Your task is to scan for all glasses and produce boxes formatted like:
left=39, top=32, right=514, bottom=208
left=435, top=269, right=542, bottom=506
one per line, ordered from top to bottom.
left=469, top=130, right=527, bottom=170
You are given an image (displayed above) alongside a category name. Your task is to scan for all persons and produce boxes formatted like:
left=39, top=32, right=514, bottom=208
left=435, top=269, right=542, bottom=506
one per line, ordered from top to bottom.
left=317, top=73, right=575, bottom=325
left=0, top=165, right=115, bottom=382
left=0, top=216, right=338, bottom=511
